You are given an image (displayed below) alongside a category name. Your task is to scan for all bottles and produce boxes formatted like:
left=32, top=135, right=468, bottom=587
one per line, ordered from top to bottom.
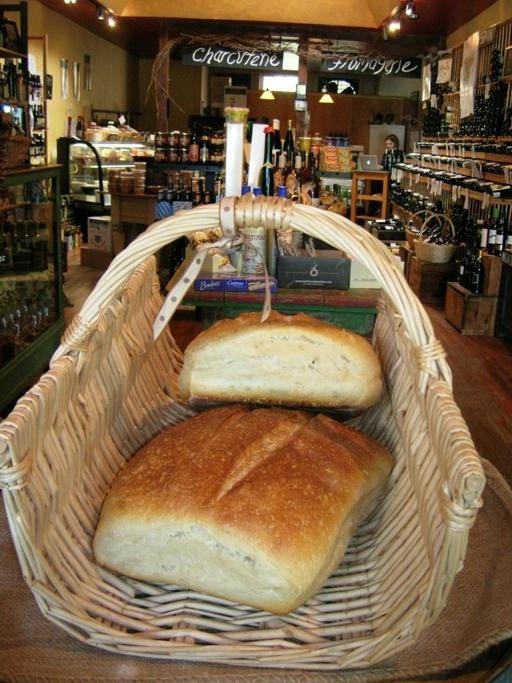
left=0, top=50, right=48, bottom=159
left=75, top=114, right=86, bottom=140
left=261, top=117, right=352, bottom=218
left=153, top=128, right=225, bottom=162
left=103, top=165, right=225, bottom=203
left=0, top=174, right=60, bottom=370
left=364, top=181, right=512, bottom=293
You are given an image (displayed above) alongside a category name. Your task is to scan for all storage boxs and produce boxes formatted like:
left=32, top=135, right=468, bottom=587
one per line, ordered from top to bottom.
left=84, top=212, right=115, bottom=254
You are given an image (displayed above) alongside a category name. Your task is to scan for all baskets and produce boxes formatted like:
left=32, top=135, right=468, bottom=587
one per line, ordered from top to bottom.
left=0, top=191, right=487, bottom=671
left=405, top=224, right=428, bottom=252
left=412, top=237, right=457, bottom=264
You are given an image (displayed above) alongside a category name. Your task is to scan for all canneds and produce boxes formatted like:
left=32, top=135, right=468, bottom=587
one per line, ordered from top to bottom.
left=154, top=130, right=192, bottom=161
left=239, top=226, right=266, bottom=276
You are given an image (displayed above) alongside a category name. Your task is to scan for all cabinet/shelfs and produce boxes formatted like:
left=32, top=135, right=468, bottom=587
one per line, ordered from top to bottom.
left=2, top=36, right=64, bottom=412
left=388, top=134, right=506, bottom=338
left=56, top=135, right=153, bottom=220
left=349, top=170, right=387, bottom=229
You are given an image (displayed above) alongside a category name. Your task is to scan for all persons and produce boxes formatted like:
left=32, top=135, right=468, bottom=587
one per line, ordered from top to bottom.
left=381, top=134, right=404, bottom=169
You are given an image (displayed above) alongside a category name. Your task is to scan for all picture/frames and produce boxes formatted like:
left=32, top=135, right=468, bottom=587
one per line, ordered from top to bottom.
left=500, top=46, right=512, bottom=77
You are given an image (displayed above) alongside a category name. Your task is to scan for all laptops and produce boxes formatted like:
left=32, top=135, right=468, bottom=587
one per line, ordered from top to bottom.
left=358, top=154, right=382, bottom=171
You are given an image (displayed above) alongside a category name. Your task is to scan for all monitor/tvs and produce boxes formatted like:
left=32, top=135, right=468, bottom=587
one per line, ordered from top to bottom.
left=2, top=103, right=24, bottom=130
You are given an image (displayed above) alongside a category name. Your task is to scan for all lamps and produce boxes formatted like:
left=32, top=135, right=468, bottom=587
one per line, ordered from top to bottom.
left=319, top=67, right=334, bottom=104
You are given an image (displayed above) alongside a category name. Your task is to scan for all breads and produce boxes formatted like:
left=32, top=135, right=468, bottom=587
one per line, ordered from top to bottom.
left=178, top=310, right=386, bottom=423
left=92, top=404, right=394, bottom=615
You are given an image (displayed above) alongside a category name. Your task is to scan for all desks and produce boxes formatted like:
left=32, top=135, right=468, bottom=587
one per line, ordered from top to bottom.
left=99, top=188, right=161, bottom=255
left=161, top=239, right=401, bottom=332
